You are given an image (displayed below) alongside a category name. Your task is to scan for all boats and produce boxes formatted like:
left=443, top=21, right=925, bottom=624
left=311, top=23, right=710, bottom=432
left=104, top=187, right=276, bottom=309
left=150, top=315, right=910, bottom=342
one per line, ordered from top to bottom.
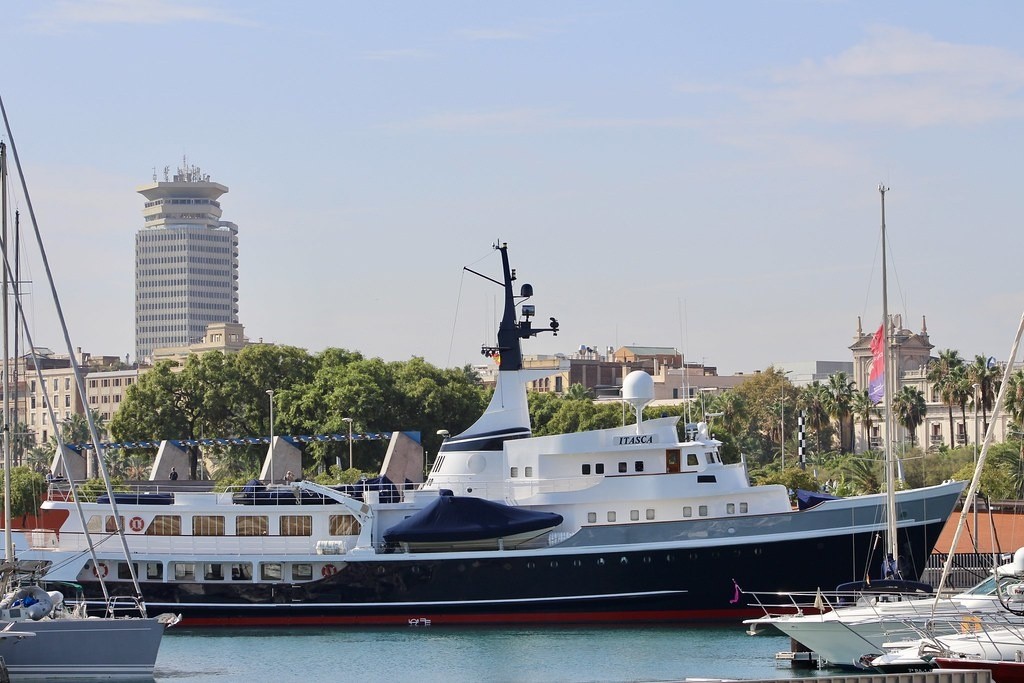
left=0, top=233, right=972, bottom=626
left=233, top=477, right=400, bottom=507
left=383, top=488, right=565, bottom=553
left=95, top=492, right=173, bottom=506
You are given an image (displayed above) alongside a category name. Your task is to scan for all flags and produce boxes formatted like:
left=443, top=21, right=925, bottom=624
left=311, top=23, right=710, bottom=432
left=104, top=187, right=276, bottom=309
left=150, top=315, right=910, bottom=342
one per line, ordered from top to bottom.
left=869, top=323, right=884, bottom=404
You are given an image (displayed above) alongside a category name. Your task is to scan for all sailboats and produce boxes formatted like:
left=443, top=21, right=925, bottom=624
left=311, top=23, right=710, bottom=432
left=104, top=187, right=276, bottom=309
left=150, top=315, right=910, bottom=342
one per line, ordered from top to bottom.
left=0, top=99, right=166, bottom=683
left=730, top=172, right=1024, bottom=683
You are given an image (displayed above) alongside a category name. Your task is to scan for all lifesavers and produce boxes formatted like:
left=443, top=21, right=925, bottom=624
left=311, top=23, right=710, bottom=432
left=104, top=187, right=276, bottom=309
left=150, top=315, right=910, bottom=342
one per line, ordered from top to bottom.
left=130, top=517, right=144, bottom=532
left=93, top=563, right=109, bottom=579
left=322, top=564, right=337, bottom=578
left=961, top=616, right=982, bottom=634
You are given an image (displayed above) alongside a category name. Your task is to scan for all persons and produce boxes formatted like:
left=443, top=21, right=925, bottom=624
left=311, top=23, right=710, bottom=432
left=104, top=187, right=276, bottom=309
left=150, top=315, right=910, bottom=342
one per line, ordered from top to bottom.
left=169, top=467, right=178, bottom=480
left=46, top=470, right=65, bottom=482
left=283, top=470, right=293, bottom=484
left=940, top=558, right=955, bottom=590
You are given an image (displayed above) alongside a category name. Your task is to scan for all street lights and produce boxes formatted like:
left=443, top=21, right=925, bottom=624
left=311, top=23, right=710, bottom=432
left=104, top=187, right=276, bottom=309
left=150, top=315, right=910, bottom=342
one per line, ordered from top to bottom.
left=266, top=388, right=275, bottom=484
left=341, top=417, right=353, bottom=469
